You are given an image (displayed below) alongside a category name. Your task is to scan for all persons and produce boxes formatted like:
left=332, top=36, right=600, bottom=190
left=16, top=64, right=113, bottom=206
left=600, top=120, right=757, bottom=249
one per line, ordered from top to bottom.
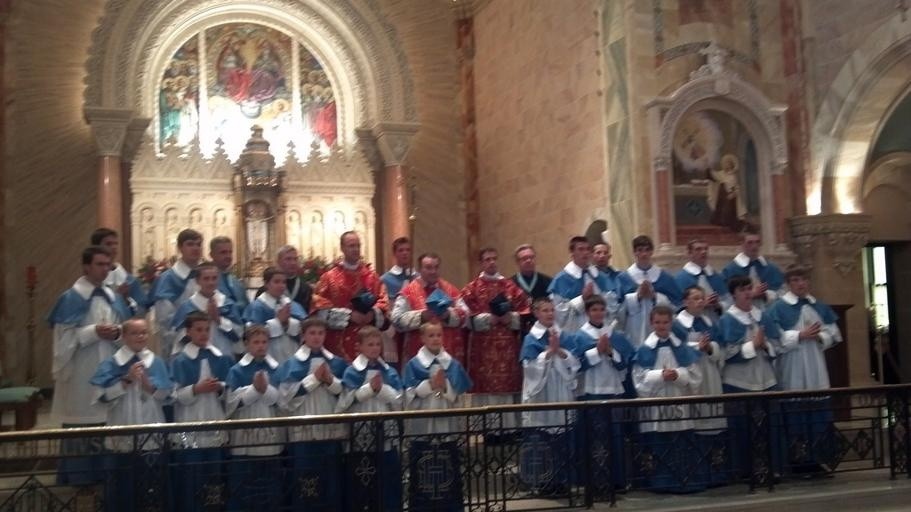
left=705, top=152, right=747, bottom=224
left=722, top=227, right=788, bottom=296
left=90, top=228, right=146, bottom=321
left=154, top=228, right=204, bottom=357
left=311, top=231, right=390, bottom=365
left=547, top=235, right=619, bottom=332
left=210, top=236, right=250, bottom=317
left=613, top=236, right=679, bottom=367
left=380, top=237, right=428, bottom=302
left=679, top=240, right=722, bottom=319
left=593, top=241, right=618, bottom=277
left=505, top=242, right=556, bottom=298
left=47, top=245, right=133, bottom=487
left=253, top=245, right=311, bottom=315
left=460, top=247, right=533, bottom=476
left=390, top=252, right=469, bottom=370
left=169, top=264, right=244, bottom=354
left=244, top=267, right=308, bottom=363
left=766, top=267, right=843, bottom=471
left=716, top=273, right=784, bottom=486
left=674, top=286, right=734, bottom=480
left=574, top=295, right=638, bottom=500
left=520, top=298, right=586, bottom=500
left=633, top=305, right=703, bottom=495
left=171, top=311, right=234, bottom=511
left=89, top=315, right=175, bottom=512
left=275, top=318, right=347, bottom=512
left=401, top=320, right=474, bottom=511
left=223, top=324, right=284, bottom=512
left=334, top=327, right=404, bottom=511
